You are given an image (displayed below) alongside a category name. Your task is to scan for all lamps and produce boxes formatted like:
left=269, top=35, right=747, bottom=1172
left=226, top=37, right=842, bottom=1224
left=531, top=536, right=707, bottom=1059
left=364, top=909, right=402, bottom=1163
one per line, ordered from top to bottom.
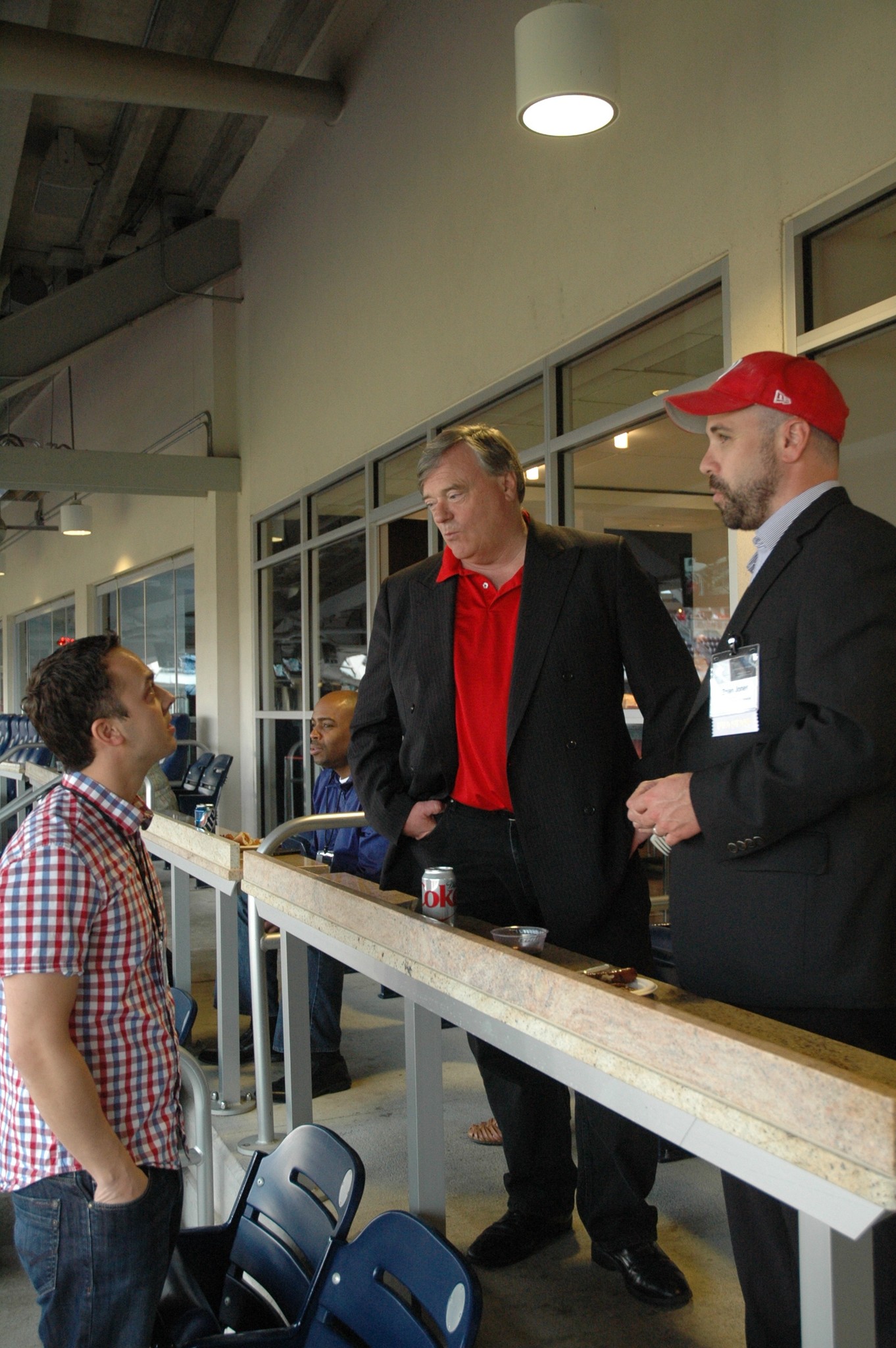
left=513, top=3, right=619, bottom=137
left=58, top=365, right=93, bottom=536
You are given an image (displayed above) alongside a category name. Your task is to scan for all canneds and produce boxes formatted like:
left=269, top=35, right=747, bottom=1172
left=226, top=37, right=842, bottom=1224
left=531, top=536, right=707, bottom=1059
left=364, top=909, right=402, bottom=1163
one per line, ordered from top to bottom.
left=195, top=804, right=216, bottom=834
left=420, top=866, right=457, bottom=929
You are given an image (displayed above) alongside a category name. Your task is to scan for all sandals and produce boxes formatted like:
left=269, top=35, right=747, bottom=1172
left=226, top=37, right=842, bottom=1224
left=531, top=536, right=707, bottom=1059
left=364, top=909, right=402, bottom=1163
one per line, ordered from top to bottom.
left=467, top=1118, right=503, bottom=1146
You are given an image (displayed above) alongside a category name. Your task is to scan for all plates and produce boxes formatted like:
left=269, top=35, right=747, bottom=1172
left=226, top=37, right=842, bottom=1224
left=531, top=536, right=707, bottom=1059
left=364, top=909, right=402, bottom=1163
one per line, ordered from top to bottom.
left=626, top=976, right=658, bottom=995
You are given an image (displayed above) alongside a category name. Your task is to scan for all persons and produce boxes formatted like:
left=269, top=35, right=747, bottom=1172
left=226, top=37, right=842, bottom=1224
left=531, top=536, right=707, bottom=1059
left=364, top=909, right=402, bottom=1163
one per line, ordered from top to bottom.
left=626, top=351, right=896, bottom=1348
left=348, top=423, right=702, bottom=1310
left=0, top=635, right=190, bottom=1348
left=198, top=690, right=392, bottom=1103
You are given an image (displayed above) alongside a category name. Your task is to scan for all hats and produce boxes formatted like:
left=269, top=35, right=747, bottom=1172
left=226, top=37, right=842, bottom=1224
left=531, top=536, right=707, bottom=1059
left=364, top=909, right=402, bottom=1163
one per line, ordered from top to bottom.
left=664, top=350, right=850, bottom=445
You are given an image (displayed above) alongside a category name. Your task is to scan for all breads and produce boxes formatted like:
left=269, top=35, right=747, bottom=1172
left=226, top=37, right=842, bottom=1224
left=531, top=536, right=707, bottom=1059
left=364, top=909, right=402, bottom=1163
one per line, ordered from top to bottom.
left=231, top=832, right=253, bottom=846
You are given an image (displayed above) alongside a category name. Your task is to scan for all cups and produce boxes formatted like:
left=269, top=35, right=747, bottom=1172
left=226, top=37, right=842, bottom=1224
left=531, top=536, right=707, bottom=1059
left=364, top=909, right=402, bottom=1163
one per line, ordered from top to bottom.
left=490, top=925, right=548, bottom=959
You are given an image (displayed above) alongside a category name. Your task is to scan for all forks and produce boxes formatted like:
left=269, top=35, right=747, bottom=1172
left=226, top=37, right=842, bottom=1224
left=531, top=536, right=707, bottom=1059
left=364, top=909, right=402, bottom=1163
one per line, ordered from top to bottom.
left=650, top=833, right=672, bottom=856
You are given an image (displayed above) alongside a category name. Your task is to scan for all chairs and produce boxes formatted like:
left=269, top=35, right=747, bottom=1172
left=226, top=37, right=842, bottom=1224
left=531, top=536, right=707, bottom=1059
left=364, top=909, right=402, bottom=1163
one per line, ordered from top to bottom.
left=0, top=714, right=55, bottom=813
left=172, top=751, right=234, bottom=817
left=155, top=1123, right=483, bottom=1348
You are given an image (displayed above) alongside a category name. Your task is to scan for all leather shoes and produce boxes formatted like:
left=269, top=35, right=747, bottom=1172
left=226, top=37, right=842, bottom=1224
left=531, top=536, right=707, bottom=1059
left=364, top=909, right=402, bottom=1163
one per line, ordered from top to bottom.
left=198, top=1025, right=277, bottom=1066
left=254, top=1055, right=352, bottom=1103
left=465, top=1210, right=575, bottom=1273
left=591, top=1230, right=692, bottom=1307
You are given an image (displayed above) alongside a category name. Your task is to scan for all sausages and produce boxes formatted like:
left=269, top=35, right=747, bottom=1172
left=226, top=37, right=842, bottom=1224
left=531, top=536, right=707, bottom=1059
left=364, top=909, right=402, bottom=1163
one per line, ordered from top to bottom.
left=584, top=967, right=638, bottom=984
